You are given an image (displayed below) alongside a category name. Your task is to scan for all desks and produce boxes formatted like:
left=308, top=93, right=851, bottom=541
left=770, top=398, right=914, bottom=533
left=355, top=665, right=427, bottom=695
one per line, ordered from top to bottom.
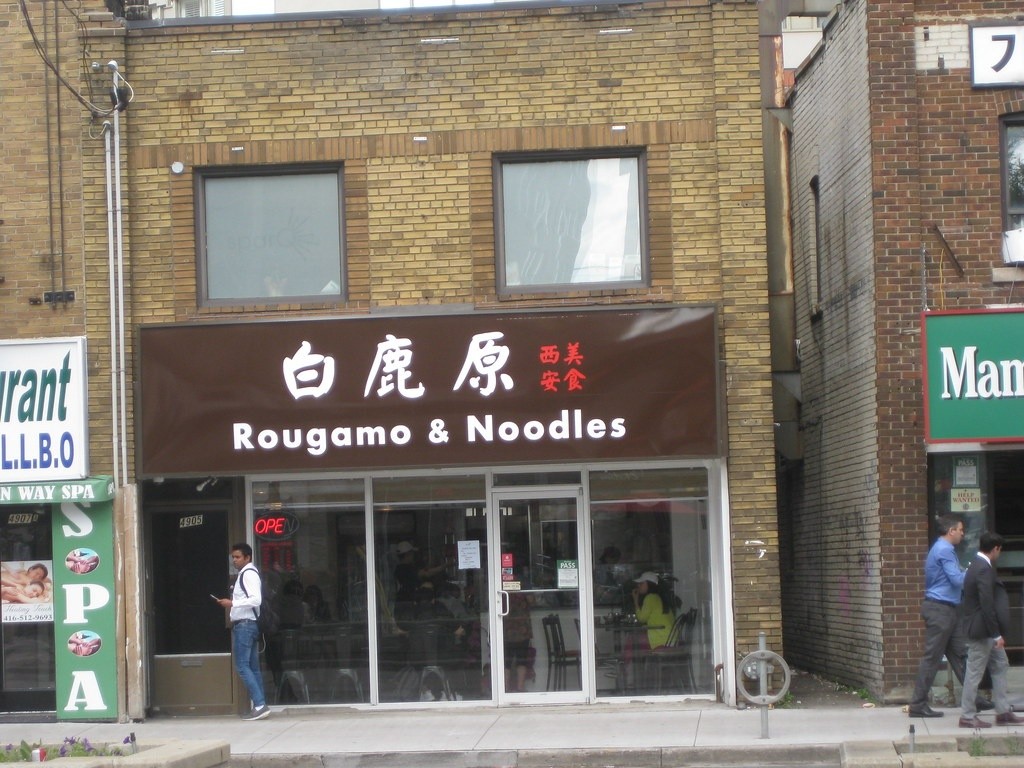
left=599, top=622, right=667, bottom=693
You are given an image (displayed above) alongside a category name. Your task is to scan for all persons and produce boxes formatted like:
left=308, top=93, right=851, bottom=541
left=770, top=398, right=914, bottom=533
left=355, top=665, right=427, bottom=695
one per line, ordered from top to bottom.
left=908, top=513, right=994, bottom=717
left=958, top=532, right=1024, bottom=728
left=392, top=542, right=481, bottom=672
left=216, top=544, right=271, bottom=721
left=595, top=547, right=633, bottom=604
left=65, top=550, right=99, bottom=574
left=1, top=562, right=52, bottom=605
left=502, top=564, right=533, bottom=693
left=612, top=571, right=680, bottom=697
left=274, top=579, right=337, bottom=672
left=68, top=633, right=101, bottom=656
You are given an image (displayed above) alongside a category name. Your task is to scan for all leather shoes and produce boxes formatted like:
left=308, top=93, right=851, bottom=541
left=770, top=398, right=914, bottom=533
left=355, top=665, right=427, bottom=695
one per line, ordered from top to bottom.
left=976, top=699, right=995, bottom=713
left=910, top=705, right=943, bottom=717
left=996, top=711, right=1024, bottom=725
left=959, top=716, right=991, bottom=728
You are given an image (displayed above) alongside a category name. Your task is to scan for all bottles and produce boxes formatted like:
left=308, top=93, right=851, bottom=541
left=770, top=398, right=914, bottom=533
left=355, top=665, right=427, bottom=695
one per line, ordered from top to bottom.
left=614, top=612, right=619, bottom=622
left=608, top=613, right=613, bottom=624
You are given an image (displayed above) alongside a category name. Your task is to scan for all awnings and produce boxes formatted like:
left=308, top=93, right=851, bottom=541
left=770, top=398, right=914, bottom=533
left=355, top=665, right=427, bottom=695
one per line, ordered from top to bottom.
left=0, top=475, right=115, bottom=505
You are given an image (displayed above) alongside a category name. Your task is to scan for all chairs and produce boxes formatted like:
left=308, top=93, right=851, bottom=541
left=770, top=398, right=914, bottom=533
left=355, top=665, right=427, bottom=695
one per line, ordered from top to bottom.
left=642, top=607, right=698, bottom=695
left=541, top=613, right=619, bottom=691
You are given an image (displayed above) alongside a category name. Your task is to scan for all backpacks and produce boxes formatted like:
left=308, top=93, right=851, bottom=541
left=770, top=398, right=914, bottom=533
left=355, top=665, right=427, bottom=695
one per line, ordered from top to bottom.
left=240, top=568, right=287, bottom=636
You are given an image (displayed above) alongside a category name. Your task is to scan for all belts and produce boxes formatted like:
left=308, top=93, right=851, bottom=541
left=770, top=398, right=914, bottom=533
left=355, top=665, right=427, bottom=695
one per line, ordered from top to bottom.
left=925, top=597, right=955, bottom=608
left=232, top=619, right=257, bottom=628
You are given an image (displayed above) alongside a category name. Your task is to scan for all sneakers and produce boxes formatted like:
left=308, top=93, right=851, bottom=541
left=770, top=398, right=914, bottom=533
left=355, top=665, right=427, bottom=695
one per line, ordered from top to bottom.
left=242, top=703, right=271, bottom=721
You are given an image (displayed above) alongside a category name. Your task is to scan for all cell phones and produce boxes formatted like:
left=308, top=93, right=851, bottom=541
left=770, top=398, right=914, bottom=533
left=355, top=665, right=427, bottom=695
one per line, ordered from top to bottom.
left=209, top=594, right=219, bottom=601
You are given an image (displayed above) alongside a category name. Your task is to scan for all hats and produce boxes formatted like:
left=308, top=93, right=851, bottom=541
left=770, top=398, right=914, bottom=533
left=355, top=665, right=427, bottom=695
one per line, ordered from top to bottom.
left=396, top=541, right=420, bottom=556
left=632, top=572, right=659, bottom=585
